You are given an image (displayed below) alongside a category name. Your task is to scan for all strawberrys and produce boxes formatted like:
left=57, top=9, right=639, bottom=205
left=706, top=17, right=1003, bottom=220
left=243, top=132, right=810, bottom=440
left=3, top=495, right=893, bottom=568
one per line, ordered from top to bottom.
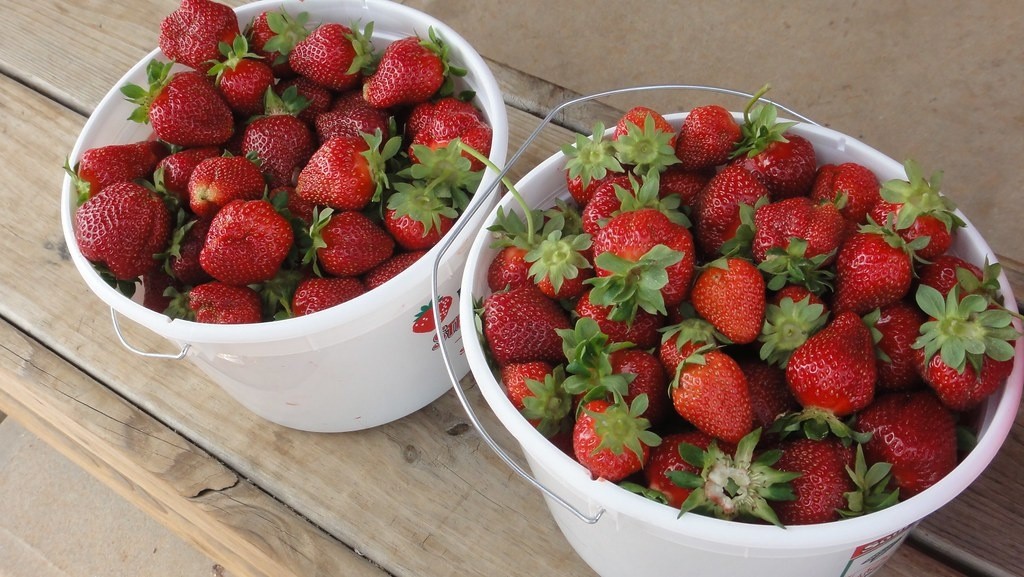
left=59, top=0, right=494, bottom=325
left=470, top=83, right=1023, bottom=528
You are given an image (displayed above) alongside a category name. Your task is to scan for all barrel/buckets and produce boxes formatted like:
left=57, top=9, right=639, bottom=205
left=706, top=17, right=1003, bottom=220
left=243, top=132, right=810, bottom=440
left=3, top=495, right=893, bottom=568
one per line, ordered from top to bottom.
left=60, top=0, right=507, bottom=433
left=430, top=84, right=1024, bottom=577
